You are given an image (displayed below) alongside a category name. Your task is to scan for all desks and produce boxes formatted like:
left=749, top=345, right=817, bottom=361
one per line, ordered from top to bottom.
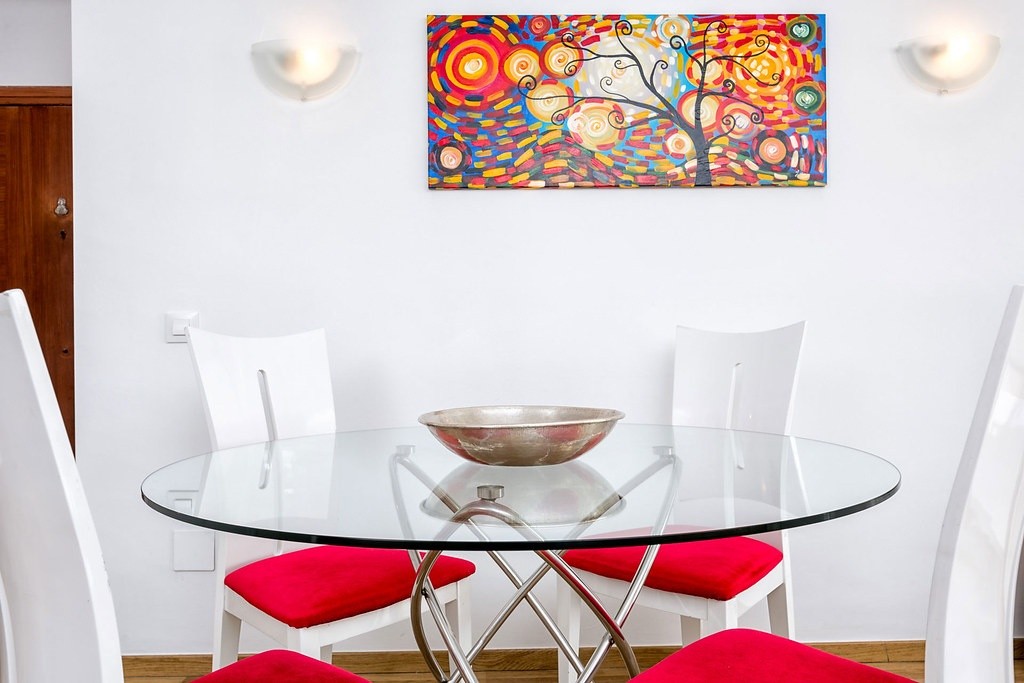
left=141, top=427, right=900, bottom=683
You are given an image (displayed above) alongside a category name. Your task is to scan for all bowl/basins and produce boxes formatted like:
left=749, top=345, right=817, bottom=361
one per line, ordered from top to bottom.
left=419, top=406, right=624, bottom=465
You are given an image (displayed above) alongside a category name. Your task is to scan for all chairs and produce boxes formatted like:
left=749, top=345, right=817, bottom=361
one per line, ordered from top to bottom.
left=629, top=286, right=1024, bottom=683
left=0, top=289, right=374, bottom=683
left=558, top=321, right=809, bottom=683
left=184, top=324, right=475, bottom=683
